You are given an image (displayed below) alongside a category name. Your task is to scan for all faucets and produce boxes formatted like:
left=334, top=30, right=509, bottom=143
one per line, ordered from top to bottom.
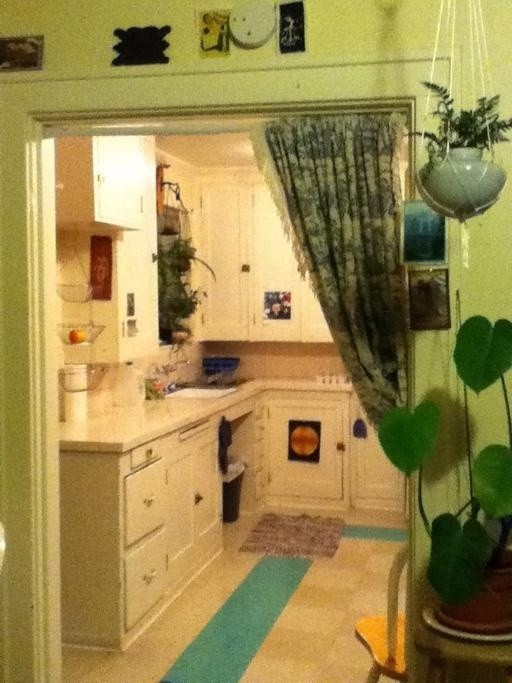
left=175, top=359, right=191, bottom=367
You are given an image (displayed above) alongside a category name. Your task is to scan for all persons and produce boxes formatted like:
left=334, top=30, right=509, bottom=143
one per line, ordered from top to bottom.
left=271, top=293, right=291, bottom=318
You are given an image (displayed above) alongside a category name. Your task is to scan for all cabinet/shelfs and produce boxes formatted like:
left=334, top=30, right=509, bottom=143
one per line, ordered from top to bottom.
left=52, top=134, right=142, bottom=233
left=201, top=173, right=336, bottom=344
left=262, top=390, right=348, bottom=525
left=348, top=391, right=408, bottom=531
left=58, top=418, right=224, bottom=655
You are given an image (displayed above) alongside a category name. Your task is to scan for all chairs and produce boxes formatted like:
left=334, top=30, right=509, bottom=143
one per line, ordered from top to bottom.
left=355, top=540, right=411, bottom=683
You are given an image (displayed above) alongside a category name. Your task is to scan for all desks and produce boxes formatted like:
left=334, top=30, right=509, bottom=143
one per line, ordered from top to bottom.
left=412, top=619, right=512, bottom=683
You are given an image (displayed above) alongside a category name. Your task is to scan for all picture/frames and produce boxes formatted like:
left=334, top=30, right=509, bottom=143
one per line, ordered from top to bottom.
left=400, top=198, right=452, bottom=331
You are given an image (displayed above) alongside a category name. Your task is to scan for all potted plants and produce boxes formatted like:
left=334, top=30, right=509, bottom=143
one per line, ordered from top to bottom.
left=399, top=78, right=512, bottom=220
left=151, top=224, right=218, bottom=319
left=376, top=312, right=512, bottom=643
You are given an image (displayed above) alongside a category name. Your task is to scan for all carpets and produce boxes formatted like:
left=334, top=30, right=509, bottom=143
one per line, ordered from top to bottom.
left=239, top=510, right=346, bottom=559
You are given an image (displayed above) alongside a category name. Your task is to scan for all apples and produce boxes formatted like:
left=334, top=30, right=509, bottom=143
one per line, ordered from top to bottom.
left=68, top=330, right=85, bottom=344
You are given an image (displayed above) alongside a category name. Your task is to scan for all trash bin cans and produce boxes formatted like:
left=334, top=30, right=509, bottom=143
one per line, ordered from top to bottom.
left=223, top=464, right=245, bottom=523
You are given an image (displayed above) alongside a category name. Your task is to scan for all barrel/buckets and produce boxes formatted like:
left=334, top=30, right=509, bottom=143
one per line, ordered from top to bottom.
left=114, top=360, right=146, bottom=407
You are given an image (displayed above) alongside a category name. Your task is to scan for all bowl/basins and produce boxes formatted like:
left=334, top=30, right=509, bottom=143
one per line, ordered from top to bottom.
left=55, top=321, right=109, bottom=348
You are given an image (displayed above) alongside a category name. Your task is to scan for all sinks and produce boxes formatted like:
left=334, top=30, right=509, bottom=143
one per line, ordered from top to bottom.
left=166, top=388, right=236, bottom=398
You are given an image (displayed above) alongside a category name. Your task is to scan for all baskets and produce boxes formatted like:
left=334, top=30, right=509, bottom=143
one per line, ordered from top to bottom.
left=58, top=283, right=92, bottom=303
left=58, top=325, right=104, bottom=346
left=59, top=364, right=109, bottom=391
